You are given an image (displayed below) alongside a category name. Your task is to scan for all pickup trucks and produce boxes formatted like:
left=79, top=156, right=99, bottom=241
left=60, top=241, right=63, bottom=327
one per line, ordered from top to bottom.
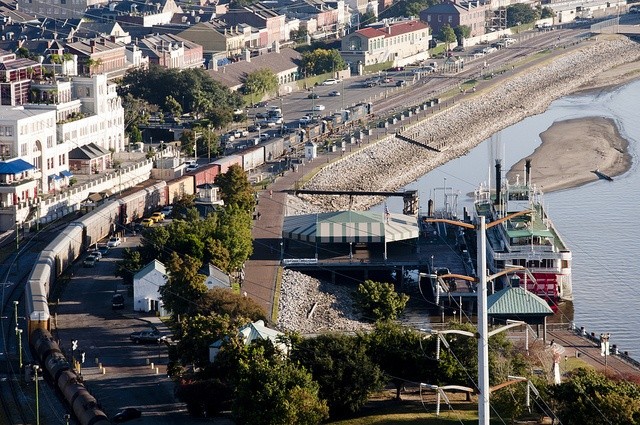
left=150, top=212, right=165, bottom=222
left=130, top=330, right=161, bottom=344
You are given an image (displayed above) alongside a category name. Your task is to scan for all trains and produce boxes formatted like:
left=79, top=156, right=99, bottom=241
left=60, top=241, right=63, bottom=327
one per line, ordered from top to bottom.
left=24, top=101, right=373, bottom=425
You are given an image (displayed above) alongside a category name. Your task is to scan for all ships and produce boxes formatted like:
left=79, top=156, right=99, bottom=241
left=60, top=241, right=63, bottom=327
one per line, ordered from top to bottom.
left=474, top=157, right=574, bottom=330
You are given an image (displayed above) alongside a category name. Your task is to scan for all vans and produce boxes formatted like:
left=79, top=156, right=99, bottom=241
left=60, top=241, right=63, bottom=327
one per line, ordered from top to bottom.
left=322, top=78, right=337, bottom=85
left=186, top=159, right=198, bottom=165
left=112, top=294, right=124, bottom=309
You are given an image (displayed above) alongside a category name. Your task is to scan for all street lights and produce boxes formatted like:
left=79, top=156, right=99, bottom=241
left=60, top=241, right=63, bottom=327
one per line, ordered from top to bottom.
left=310, top=87, right=314, bottom=116
left=207, top=125, right=211, bottom=162
left=150, top=137, right=152, bottom=151
left=160, top=141, right=164, bottom=181
left=15, top=221, right=20, bottom=249
left=13, top=301, right=19, bottom=335
left=18, top=329, right=23, bottom=368
left=33, top=365, right=43, bottom=425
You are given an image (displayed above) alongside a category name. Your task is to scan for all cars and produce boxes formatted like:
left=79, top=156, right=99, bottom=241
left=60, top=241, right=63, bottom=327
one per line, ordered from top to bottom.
left=331, top=90, right=340, bottom=95
left=224, top=105, right=283, bottom=139
left=314, top=105, right=325, bottom=110
left=299, top=115, right=310, bottom=123
left=186, top=162, right=199, bottom=171
left=161, top=206, right=173, bottom=216
left=139, top=219, right=153, bottom=227
left=108, top=237, right=121, bottom=247
left=98, top=247, right=109, bottom=255
left=87, top=252, right=101, bottom=260
left=84, top=257, right=95, bottom=266
